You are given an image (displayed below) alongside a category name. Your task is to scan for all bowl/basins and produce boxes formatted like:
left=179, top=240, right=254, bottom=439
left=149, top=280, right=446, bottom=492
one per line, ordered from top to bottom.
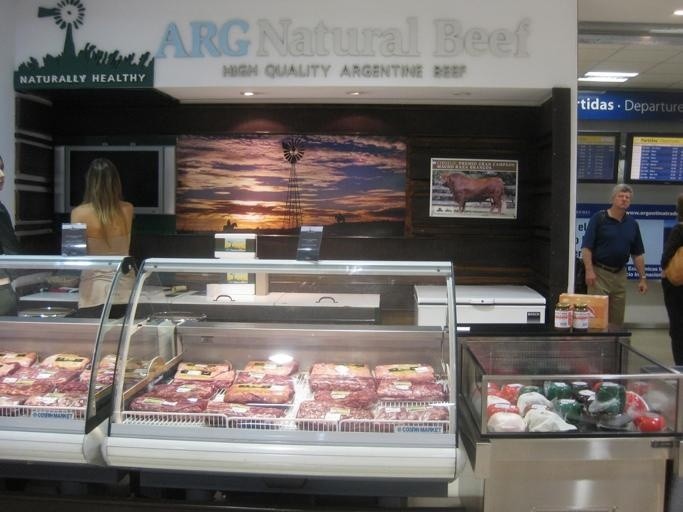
left=46, top=274, right=80, bottom=289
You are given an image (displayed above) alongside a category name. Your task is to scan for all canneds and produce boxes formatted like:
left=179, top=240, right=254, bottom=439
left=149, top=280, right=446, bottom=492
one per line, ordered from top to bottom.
left=553, top=302, right=572, bottom=332
left=573, top=302, right=588, bottom=332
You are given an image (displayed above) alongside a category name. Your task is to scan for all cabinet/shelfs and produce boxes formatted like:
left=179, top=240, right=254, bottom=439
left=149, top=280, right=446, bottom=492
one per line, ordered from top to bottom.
left=0, top=253, right=154, bottom=511
left=106, top=258, right=459, bottom=510
left=458, top=339, right=683, bottom=512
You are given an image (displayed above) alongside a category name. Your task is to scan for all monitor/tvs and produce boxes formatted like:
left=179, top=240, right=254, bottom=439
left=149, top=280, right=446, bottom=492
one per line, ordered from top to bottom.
left=576, top=128, right=621, bottom=184
left=623, top=131, right=682, bottom=186
left=53, top=144, right=176, bottom=215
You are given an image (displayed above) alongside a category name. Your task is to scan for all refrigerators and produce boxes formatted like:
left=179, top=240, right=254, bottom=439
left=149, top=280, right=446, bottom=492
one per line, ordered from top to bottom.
left=416, top=282, right=549, bottom=330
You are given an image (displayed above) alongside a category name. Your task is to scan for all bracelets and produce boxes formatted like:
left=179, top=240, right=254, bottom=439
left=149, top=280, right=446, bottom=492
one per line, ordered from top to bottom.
left=640, top=276, right=646, bottom=279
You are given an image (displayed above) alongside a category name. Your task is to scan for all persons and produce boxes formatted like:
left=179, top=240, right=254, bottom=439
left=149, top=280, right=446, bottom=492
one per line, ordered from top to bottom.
left=0, top=156, right=22, bottom=317
left=71, top=159, right=137, bottom=320
left=583, top=184, right=648, bottom=371
left=661, top=198, right=683, bottom=368
